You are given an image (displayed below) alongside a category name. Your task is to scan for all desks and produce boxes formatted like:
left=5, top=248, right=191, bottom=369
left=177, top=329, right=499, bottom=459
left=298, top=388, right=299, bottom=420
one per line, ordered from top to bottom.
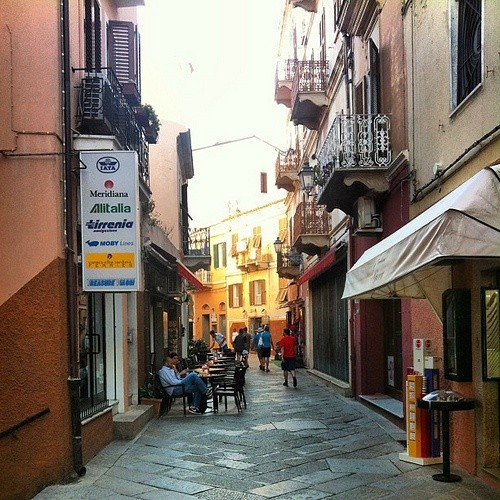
left=186, top=352, right=236, bottom=414
left=417, top=395, right=476, bottom=482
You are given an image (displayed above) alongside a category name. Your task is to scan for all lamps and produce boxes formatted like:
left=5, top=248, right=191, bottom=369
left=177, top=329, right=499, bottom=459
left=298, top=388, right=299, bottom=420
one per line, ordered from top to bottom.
left=297, top=162, right=319, bottom=197
left=273, top=237, right=282, bottom=253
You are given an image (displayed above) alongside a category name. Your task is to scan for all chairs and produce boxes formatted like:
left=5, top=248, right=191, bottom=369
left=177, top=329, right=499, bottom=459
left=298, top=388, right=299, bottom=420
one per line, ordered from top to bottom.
left=156, top=349, right=248, bottom=418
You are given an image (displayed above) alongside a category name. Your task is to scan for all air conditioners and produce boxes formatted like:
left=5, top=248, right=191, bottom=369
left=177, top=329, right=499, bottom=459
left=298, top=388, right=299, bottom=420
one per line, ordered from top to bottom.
left=82, top=73, right=114, bottom=120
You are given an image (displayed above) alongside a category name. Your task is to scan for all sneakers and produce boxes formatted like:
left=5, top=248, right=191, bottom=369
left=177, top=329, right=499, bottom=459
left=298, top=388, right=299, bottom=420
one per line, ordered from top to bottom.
left=206, top=385, right=220, bottom=398
left=189, top=405, right=201, bottom=415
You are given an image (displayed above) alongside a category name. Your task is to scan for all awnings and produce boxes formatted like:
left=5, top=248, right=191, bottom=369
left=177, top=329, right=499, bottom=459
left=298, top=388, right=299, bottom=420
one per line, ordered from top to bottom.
left=341, top=163, right=500, bottom=325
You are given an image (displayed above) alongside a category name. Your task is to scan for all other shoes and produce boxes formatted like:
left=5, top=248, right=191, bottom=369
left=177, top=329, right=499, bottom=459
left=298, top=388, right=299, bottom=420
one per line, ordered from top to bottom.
left=260, top=365, right=270, bottom=372
left=293, top=378, right=297, bottom=387
left=283, top=381, right=288, bottom=386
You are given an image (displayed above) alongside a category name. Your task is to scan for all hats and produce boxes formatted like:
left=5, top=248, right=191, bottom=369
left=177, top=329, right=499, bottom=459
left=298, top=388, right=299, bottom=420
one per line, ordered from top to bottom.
left=256, top=327, right=264, bottom=332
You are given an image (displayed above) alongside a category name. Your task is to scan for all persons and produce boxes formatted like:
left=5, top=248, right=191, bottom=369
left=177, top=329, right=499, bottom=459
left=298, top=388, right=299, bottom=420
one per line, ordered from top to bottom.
left=261, top=325, right=275, bottom=372
left=243, top=327, right=251, bottom=354
left=232, top=328, right=239, bottom=342
left=253, top=328, right=264, bottom=370
left=279, top=328, right=297, bottom=387
left=233, top=329, right=247, bottom=361
left=209, top=330, right=228, bottom=353
left=251, top=330, right=258, bottom=351
left=159, top=352, right=219, bottom=415
